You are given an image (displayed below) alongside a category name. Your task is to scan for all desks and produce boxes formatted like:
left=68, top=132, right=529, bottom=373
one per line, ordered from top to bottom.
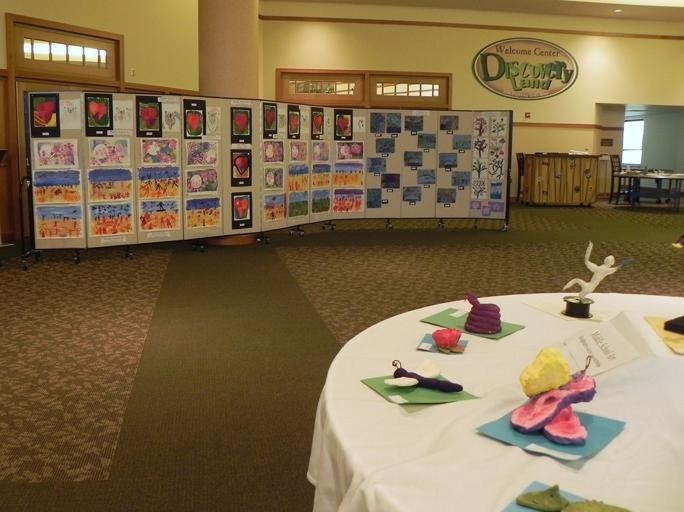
left=614, top=171, right=684, bottom=214
left=306, top=291, right=684, bottom=512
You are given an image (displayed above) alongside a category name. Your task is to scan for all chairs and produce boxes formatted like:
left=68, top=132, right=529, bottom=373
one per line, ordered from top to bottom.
left=516, top=153, right=525, bottom=203
left=609, top=155, right=625, bottom=204
